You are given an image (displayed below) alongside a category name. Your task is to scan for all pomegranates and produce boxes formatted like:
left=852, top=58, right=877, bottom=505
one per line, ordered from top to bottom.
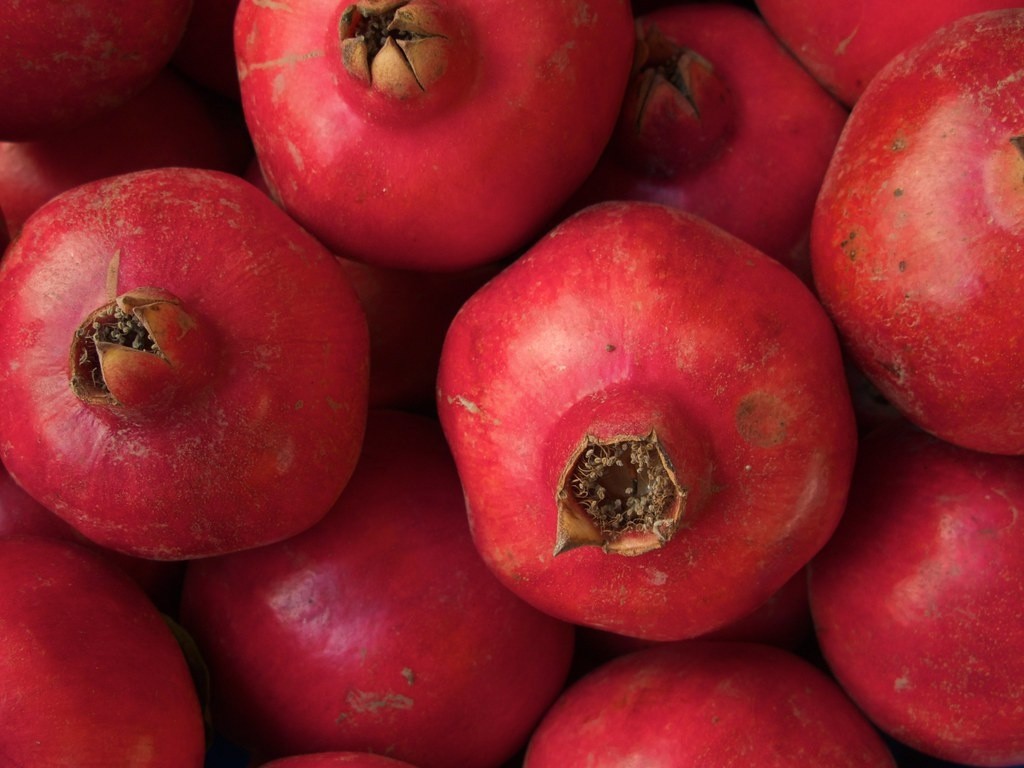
left=1, top=0, right=1024, bottom=768
